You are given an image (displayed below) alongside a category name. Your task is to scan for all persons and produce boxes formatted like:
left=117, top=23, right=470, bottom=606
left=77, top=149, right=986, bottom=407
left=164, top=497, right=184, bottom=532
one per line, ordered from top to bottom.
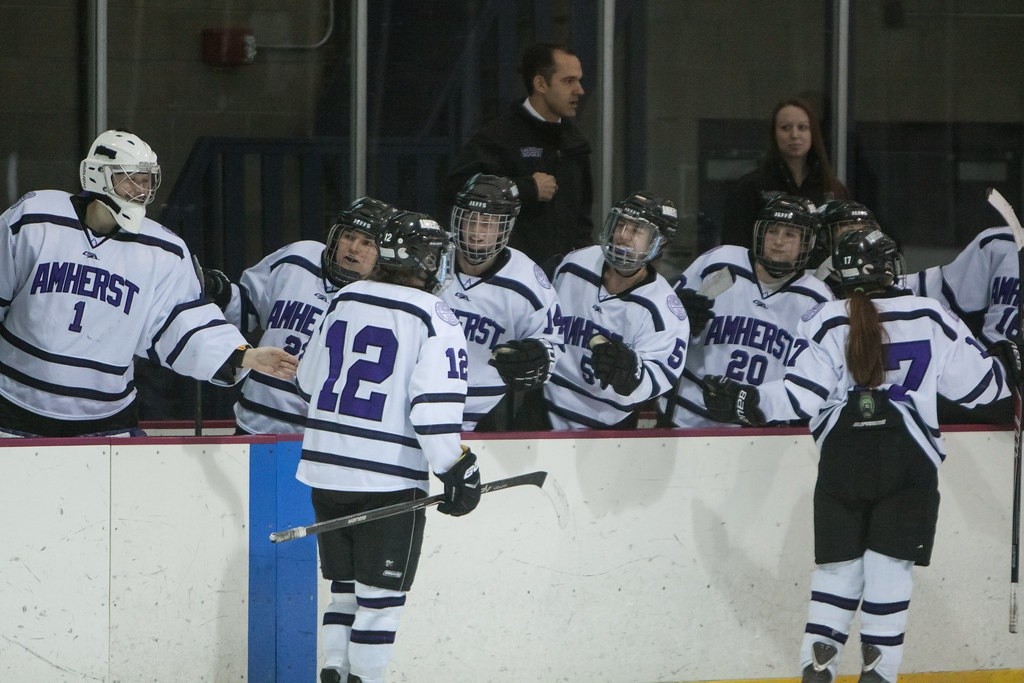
left=441, top=44, right=595, bottom=282
left=721, top=98, right=851, bottom=269
left=0, top=129, right=300, bottom=439
left=434, top=173, right=564, bottom=433
left=512, top=191, right=690, bottom=432
left=655, top=195, right=835, bottom=428
left=192, top=197, right=398, bottom=435
left=805, top=199, right=1024, bottom=428
left=295, top=210, right=481, bottom=683
left=703, top=231, right=1024, bottom=683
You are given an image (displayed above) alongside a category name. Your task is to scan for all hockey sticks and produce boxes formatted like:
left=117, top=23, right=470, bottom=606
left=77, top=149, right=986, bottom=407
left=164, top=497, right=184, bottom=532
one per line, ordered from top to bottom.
left=987, top=187, right=1024, bottom=634
left=192, top=254, right=204, bottom=436
left=659, top=263, right=737, bottom=427
left=587, top=333, right=613, bottom=350
left=491, top=343, right=526, bottom=432
left=269, top=470, right=549, bottom=544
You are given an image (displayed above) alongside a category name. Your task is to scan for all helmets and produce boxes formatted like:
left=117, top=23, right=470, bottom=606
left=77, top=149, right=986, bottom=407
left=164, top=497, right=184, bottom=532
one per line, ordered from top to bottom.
left=79, top=129, right=162, bottom=235
left=449, top=173, right=521, bottom=266
left=598, top=191, right=678, bottom=278
left=753, top=193, right=821, bottom=279
left=321, top=197, right=399, bottom=289
left=816, top=200, right=882, bottom=253
left=375, top=209, right=456, bottom=295
left=832, top=226, right=908, bottom=288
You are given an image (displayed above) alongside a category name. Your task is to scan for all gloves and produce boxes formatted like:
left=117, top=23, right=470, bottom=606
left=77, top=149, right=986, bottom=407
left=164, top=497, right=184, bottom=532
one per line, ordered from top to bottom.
left=194, top=253, right=232, bottom=309
left=675, top=287, right=716, bottom=338
left=488, top=336, right=556, bottom=392
left=591, top=339, right=645, bottom=396
left=985, top=340, right=1023, bottom=389
left=703, top=374, right=767, bottom=429
left=433, top=445, right=481, bottom=517
